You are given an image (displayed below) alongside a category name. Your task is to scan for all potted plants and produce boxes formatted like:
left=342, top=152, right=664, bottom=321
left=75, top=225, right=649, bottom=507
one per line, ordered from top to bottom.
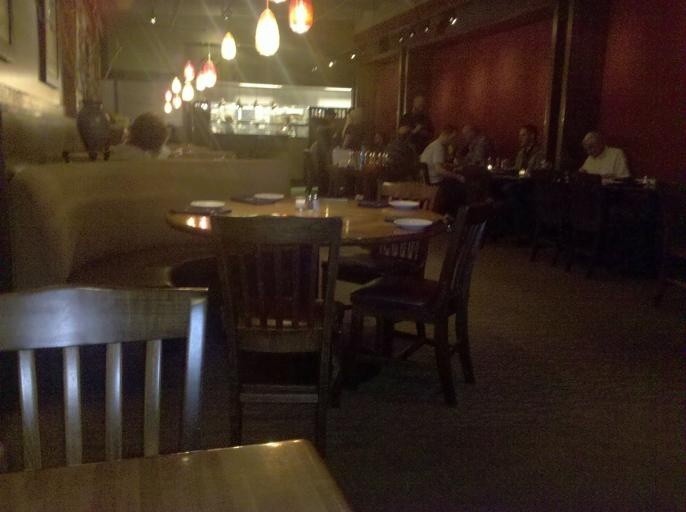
left=58, top=21, right=124, bottom=160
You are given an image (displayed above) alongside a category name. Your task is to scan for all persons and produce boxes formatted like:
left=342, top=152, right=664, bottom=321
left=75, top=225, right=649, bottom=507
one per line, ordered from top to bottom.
left=304, top=96, right=514, bottom=225
left=110, top=114, right=167, bottom=159
left=502, top=124, right=545, bottom=173
left=578, top=132, right=631, bottom=185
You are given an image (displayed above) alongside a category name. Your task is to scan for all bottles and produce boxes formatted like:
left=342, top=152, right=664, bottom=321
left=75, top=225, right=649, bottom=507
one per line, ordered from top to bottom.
left=308, top=194, right=319, bottom=211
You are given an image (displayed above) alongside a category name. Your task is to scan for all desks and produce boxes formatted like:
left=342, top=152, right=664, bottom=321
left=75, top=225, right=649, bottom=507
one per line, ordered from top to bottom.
left=0, top=438, right=352, bottom=512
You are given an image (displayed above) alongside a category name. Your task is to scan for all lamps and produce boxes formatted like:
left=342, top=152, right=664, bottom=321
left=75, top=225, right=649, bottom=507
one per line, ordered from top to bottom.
left=163, top=0, right=314, bottom=113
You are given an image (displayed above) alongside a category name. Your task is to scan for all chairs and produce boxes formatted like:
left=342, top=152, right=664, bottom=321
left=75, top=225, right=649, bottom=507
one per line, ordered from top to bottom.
left=300, top=145, right=686, bottom=310
left=347, top=198, right=504, bottom=409
left=211, top=211, right=342, bottom=449
left=2, top=278, right=212, bottom=472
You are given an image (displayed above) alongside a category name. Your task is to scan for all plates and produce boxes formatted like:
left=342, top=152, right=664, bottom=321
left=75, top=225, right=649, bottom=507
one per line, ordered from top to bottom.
left=254, top=193, right=285, bottom=200
left=190, top=200, right=225, bottom=208
left=389, top=200, right=419, bottom=207
left=394, top=217, right=432, bottom=229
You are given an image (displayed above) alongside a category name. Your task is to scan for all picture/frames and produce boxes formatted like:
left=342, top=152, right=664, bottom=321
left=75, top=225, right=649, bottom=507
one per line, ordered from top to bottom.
left=0, top=0, right=16, bottom=63
left=38, top=0, right=61, bottom=88
